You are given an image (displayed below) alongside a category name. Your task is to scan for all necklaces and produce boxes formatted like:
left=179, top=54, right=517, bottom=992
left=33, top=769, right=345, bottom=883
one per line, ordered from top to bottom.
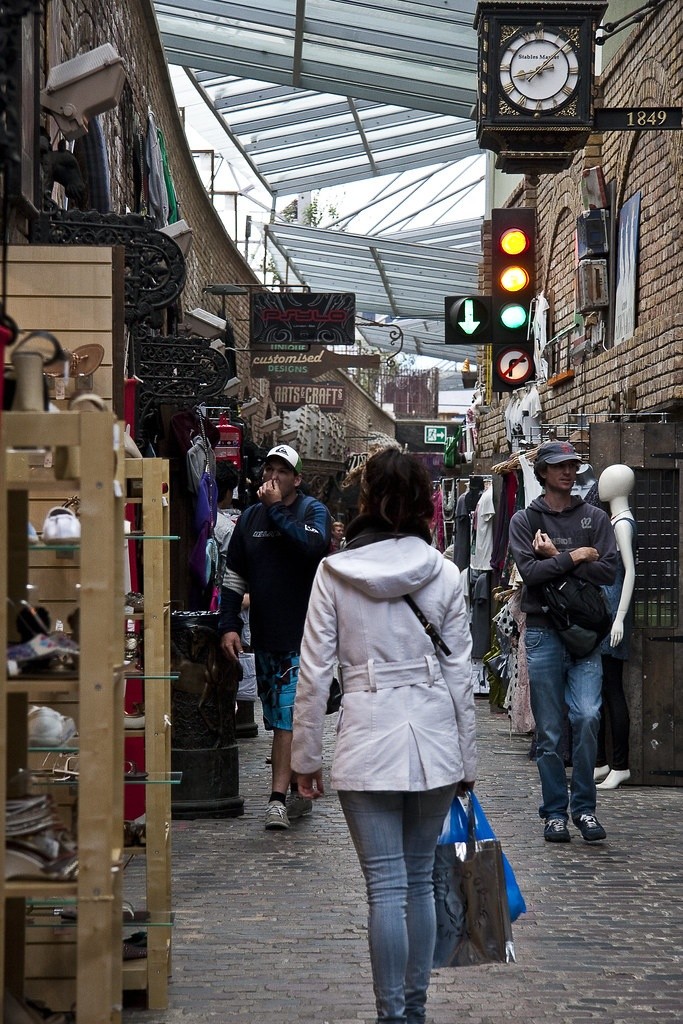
left=609, top=507, right=630, bottom=521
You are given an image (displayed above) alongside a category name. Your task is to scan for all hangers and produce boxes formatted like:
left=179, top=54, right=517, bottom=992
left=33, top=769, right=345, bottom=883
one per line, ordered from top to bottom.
left=491, top=430, right=589, bottom=476
left=492, top=585, right=516, bottom=603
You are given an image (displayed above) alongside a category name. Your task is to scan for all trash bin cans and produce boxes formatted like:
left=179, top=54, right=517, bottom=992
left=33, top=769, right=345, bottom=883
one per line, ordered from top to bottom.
left=170, top=610, right=244, bottom=821
left=235, top=653, right=258, bottom=738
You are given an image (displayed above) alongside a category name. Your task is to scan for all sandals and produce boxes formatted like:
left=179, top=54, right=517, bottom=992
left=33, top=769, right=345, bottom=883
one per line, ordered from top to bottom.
left=124, top=702, right=145, bottom=729
left=6, top=794, right=119, bottom=881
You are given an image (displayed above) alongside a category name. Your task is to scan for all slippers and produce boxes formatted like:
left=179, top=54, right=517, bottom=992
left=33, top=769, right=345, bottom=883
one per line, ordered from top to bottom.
left=18, top=751, right=150, bottom=784
left=59, top=901, right=150, bottom=923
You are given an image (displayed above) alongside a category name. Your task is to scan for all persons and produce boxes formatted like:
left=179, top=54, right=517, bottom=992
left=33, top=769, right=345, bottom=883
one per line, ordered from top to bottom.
left=509, top=441, right=626, bottom=843
left=220, top=445, right=330, bottom=829
left=291, top=450, right=479, bottom=1024
left=213, top=459, right=249, bottom=611
left=589, top=465, right=637, bottom=791
left=328, top=523, right=347, bottom=552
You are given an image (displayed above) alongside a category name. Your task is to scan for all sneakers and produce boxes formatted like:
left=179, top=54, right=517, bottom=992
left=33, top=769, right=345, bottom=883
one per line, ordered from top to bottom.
left=265, top=791, right=312, bottom=828
left=571, top=810, right=607, bottom=841
left=544, top=817, right=571, bottom=843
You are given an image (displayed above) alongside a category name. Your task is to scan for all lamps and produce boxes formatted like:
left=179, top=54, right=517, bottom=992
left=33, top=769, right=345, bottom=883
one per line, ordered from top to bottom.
left=39, top=42, right=128, bottom=141
left=156, top=218, right=193, bottom=259
left=202, top=283, right=248, bottom=296
left=177, top=307, right=228, bottom=340
left=210, top=338, right=226, bottom=355
left=223, top=377, right=241, bottom=398
left=240, top=396, right=260, bottom=419
left=255, top=416, right=282, bottom=434
left=276, top=427, right=299, bottom=441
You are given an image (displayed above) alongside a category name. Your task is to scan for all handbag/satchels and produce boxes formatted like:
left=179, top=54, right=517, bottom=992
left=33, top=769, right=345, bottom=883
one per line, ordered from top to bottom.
left=538, top=573, right=612, bottom=659
left=432, top=789, right=527, bottom=969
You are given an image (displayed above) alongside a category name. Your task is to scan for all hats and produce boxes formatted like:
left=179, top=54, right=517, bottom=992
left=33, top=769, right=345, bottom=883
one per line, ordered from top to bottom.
left=533, top=441, right=583, bottom=464
left=267, top=445, right=302, bottom=474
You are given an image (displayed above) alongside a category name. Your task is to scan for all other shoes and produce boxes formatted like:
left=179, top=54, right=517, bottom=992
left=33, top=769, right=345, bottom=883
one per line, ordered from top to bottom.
left=40, top=507, right=80, bottom=544
left=27, top=703, right=75, bottom=746
left=124, top=820, right=169, bottom=847
left=123, top=931, right=147, bottom=959
left=25, top=996, right=78, bottom=1024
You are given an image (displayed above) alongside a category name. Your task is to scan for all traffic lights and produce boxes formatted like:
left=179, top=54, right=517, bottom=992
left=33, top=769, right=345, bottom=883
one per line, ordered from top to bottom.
left=491, top=207, right=536, bottom=345
left=444, top=295, right=491, bottom=344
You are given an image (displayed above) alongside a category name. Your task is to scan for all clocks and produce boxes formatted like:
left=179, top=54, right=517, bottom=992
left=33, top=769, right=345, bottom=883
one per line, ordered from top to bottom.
left=473, top=0, right=609, bottom=174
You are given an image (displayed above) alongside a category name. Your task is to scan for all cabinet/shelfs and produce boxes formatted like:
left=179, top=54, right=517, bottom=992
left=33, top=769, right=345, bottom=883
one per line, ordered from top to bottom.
left=1, top=408, right=184, bottom=1024
